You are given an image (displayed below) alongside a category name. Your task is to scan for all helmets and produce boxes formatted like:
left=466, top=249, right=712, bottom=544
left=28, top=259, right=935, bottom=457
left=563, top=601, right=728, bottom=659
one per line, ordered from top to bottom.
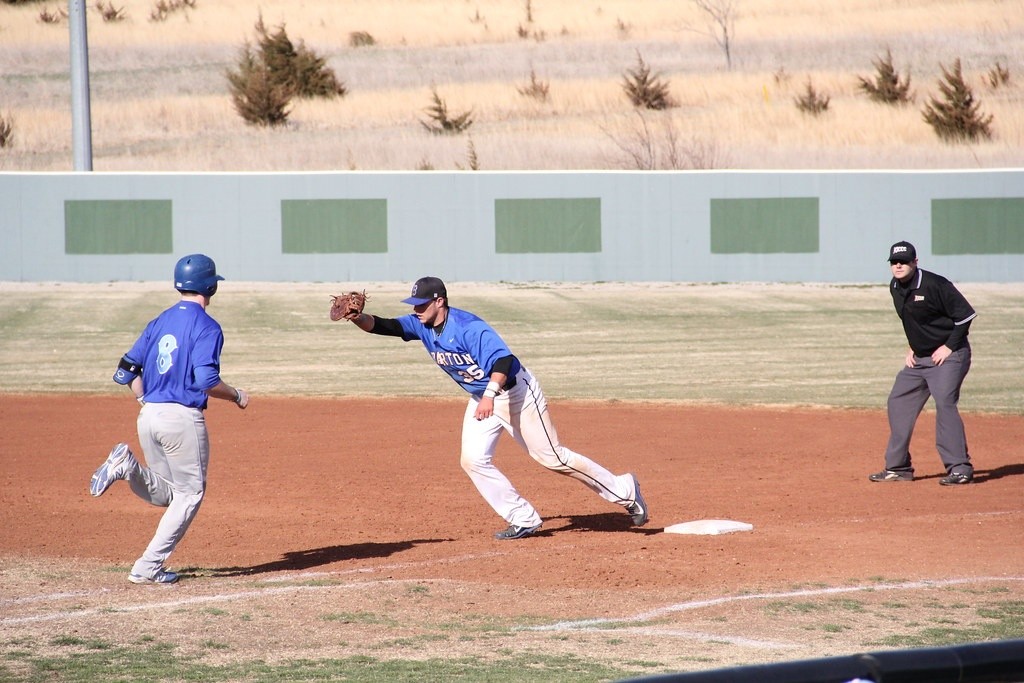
left=175, top=254, right=225, bottom=298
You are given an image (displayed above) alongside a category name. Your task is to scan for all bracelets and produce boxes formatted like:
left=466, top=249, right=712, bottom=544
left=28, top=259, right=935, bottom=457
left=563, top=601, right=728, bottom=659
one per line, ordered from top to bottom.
left=229, top=388, right=241, bottom=402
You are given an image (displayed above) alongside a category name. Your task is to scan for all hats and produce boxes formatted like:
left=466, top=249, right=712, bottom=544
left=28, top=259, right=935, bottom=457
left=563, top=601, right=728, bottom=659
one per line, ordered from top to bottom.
left=887, top=241, right=916, bottom=263
left=400, top=276, right=448, bottom=306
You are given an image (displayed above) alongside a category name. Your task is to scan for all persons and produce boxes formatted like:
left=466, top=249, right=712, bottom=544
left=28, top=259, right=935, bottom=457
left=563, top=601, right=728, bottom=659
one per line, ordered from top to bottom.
left=868, top=240, right=977, bottom=486
left=89, top=254, right=248, bottom=584
left=346, top=276, right=648, bottom=540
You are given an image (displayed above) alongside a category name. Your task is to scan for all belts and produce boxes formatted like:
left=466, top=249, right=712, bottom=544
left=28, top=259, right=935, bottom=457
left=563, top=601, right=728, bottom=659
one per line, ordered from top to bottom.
left=496, top=365, right=525, bottom=396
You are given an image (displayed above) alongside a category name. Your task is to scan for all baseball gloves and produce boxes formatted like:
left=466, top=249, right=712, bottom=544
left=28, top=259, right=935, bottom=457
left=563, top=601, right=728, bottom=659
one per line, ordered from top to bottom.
left=328, top=288, right=373, bottom=323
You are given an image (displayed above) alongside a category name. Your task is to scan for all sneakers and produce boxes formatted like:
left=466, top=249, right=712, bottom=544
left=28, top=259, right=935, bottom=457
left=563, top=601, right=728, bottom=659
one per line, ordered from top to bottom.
left=90, top=442, right=136, bottom=498
left=869, top=470, right=913, bottom=481
left=624, top=472, right=649, bottom=526
left=939, top=472, right=971, bottom=485
left=497, top=523, right=543, bottom=539
left=129, top=566, right=178, bottom=584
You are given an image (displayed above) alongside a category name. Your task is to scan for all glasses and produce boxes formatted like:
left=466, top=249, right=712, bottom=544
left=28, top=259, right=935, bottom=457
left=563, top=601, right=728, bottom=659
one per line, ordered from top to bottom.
left=890, top=258, right=913, bottom=265
